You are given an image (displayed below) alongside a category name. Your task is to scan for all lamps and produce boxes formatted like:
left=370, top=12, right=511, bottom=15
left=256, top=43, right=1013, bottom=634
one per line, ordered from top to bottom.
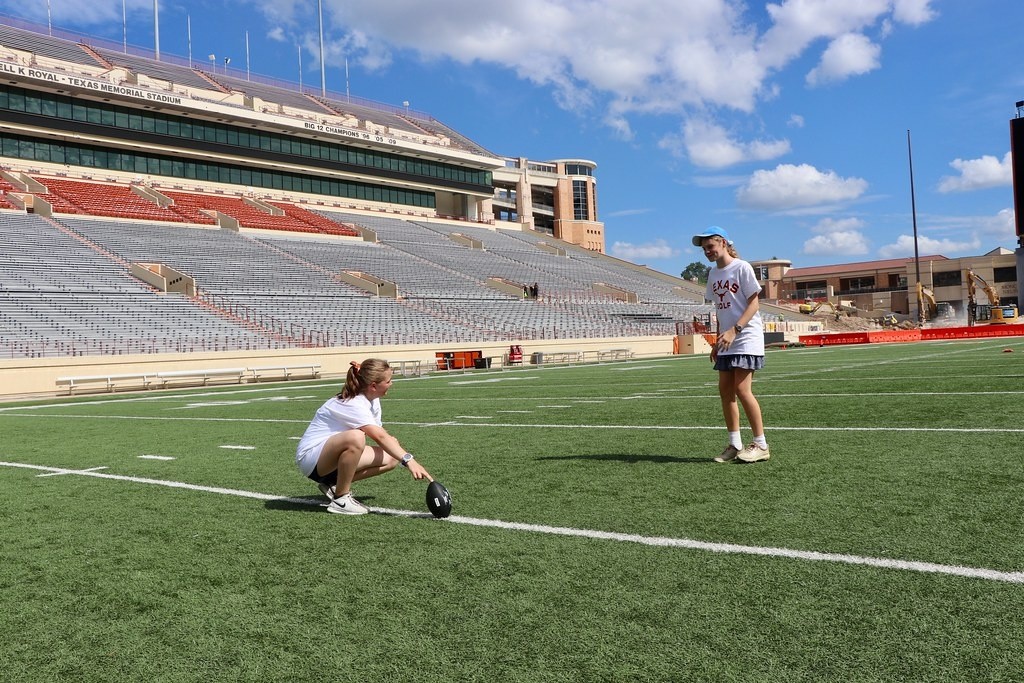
left=209, top=54, right=216, bottom=73
left=224, top=57, right=230, bottom=75
left=403, top=101, right=409, bottom=115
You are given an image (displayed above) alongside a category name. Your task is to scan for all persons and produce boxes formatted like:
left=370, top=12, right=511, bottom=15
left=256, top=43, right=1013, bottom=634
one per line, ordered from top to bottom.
left=692, top=226, right=771, bottom=464
left=533, top=283, right=538, bottom=300
left=523, top=285, right=528, bottom=299
left=530, top=286, right=534, bottom=299
left=779, top=312, right=784, bottom=321
left=296, top=358, right=433, bottom=515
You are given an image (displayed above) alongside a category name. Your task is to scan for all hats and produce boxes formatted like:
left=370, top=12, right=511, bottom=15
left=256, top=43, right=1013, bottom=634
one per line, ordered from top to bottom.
left=692, top=226, right=733, bottom=246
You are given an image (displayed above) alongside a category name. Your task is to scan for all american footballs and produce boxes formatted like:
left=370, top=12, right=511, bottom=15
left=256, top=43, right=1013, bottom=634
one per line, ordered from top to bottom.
left=426, top=480, right=452, bottom=518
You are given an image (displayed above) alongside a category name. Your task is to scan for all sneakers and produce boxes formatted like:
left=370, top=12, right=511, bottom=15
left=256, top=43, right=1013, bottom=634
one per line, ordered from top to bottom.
left=737, top=441, right=770, bottom=462
left=713, top=445, right=746, bottom=462
left=318, top=483, right=337, bottom=500
left=327, top=492, right=370, bottom=515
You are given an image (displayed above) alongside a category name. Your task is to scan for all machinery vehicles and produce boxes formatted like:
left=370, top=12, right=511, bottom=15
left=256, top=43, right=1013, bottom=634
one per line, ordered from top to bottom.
left=963, top=267, right=1019, bottom=325
left=914, top=284, right=960, bottom=330
left=800, top=295, right=847, bottom=323
left=877, top=314, right=914, bottom=330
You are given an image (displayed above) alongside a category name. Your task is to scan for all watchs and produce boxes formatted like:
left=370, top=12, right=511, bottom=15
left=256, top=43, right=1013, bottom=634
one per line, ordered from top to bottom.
left=735, top=324, right=742, bottom=332
left=402, top=453, right=413, bottom=467
